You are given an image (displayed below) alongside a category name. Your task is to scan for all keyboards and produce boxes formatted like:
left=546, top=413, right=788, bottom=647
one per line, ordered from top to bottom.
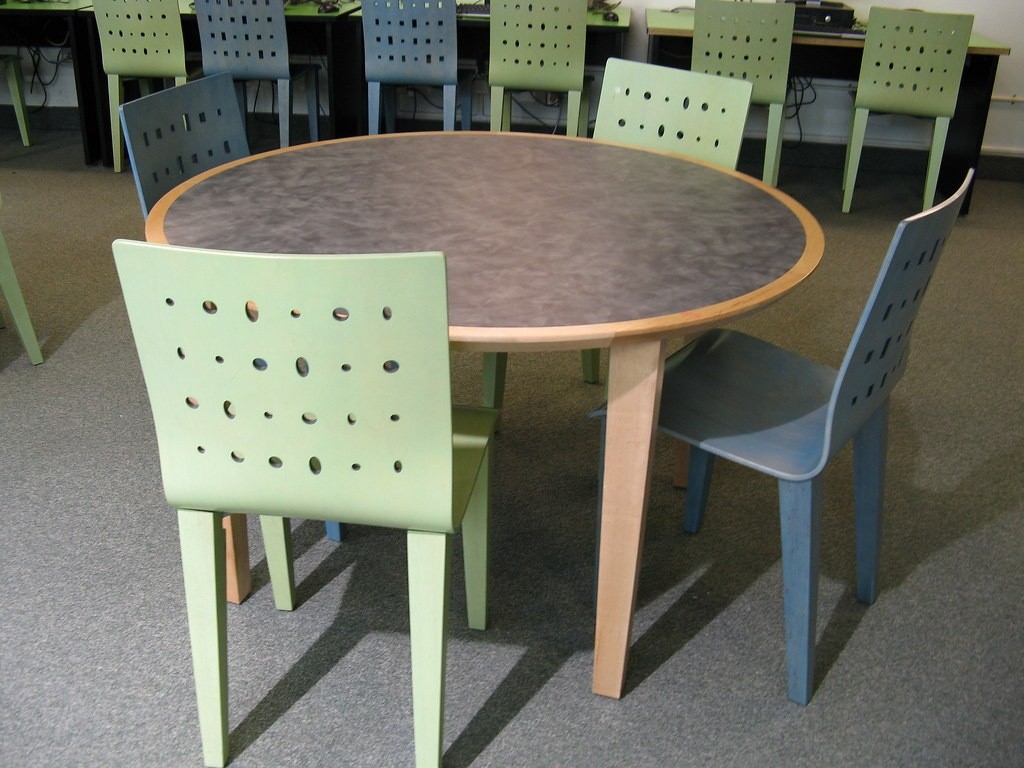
left=455, top=4, right=490, bottom=17
left=792, top=19, right=866, bottom=41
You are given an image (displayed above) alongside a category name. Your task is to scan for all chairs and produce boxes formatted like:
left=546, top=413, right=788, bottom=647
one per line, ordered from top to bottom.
left=92, top=0, right=974, bottom=213
left=0, top=52, right=32, bottom=147
left=482, top=58, right=754, bottom=435
left=116, top=71, right=353, bottom=544
left=584, top=165, right=977, bottom=708
left=110, top=239, right=504, bottom=768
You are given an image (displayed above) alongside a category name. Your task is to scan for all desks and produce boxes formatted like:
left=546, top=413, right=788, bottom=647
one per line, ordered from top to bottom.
left=0, top=0, right=101, bottom=167
left=76, top=0, right=361, bottom=168
left=346, top=0, right=633, bottom=138
left=643, top=7, right=1012, bottom=217
left=143, top=130, right=825, bottom=701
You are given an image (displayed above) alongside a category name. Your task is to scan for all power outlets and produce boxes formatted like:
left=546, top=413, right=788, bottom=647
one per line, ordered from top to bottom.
left=62, top=48, right=73, bottom=64
left=545, top=92, right=560, bottom=108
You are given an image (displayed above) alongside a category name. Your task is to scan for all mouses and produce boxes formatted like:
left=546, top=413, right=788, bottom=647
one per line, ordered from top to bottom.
left=318, top=3, right=339, bottom=14
left=603, top=11, right=618, bottom=22
